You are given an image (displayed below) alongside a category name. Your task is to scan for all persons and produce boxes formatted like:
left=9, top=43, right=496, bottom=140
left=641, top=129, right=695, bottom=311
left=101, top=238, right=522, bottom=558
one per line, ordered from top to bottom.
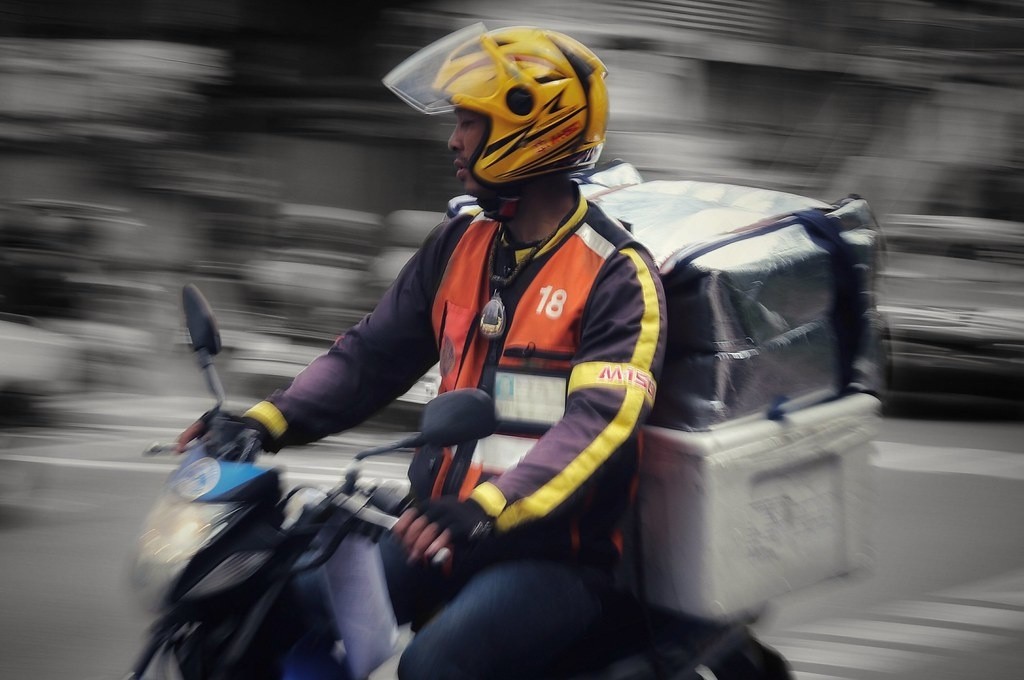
left=179, top=23, right=665, bottom=680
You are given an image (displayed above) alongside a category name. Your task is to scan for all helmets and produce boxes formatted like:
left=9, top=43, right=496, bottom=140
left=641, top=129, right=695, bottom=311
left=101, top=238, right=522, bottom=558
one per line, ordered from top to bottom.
left=437, top=22, right=612, bottom=183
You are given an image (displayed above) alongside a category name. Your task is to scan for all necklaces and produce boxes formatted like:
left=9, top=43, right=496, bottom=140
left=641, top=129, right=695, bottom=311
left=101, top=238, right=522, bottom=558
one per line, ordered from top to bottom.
left=478, top=224, right=557, bottom=339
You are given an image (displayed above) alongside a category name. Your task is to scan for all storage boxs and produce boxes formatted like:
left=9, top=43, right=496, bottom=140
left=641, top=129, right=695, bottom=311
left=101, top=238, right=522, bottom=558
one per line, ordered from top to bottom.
left=577, top=162, right=879, bottom=433
left=635, top=391, right=884, bottom=619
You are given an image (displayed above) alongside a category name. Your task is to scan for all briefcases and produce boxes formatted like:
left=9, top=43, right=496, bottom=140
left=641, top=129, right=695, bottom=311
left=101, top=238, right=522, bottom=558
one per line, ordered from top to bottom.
left=592, top=176, right=887, bottom=434
